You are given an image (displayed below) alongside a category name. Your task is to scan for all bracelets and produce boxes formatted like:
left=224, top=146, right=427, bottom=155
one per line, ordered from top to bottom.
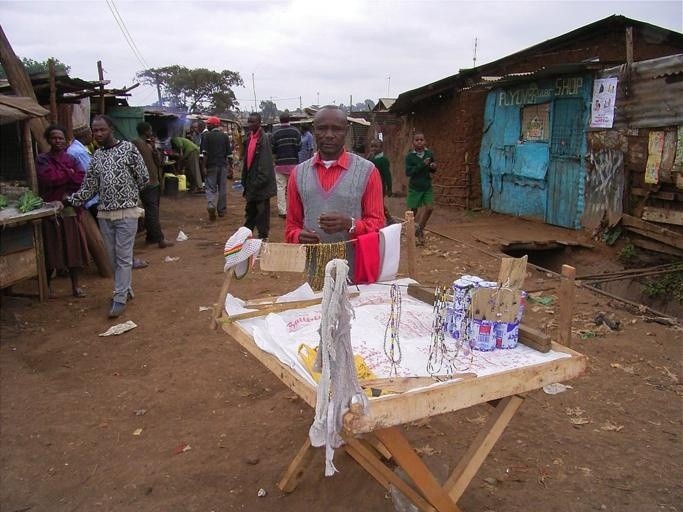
left=347, top=216, right=357, bottom=237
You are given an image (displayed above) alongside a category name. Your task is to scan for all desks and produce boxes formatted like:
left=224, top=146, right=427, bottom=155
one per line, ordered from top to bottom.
left=0, top=200, right=64, bottom=304
left=215, top=278, right=588, bottom=512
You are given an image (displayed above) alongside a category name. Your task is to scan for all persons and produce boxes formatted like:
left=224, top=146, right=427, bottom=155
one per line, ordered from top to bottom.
left=285, top=104, right=386, bottom=285
left=241, top=112, right=312, bottom=240
left=61, top=115, right=150, bottom=316
left=173, top=116, right=233, bottom=220
left=38, top=121, right=149, bottom=279
left=128, top=121, right=175, bottom=248
left=404, top=130, right=437, bottom=245
left=366, top=137, right=398, bottom=226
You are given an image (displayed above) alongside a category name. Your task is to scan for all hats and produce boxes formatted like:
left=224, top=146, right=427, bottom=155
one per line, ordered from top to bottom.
left=203, top=117, right=220, bottom=125
left=72, top=123, right=90, bottom=137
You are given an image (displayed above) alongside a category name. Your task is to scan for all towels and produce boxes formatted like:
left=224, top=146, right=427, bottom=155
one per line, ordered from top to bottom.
left=259, top=223, right=403, bottom=294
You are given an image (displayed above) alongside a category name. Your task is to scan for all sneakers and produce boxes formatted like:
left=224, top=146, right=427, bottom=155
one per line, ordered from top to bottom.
left=209, top=205, right=216, bottom=221
left=73, top=288, right=86, bottom=297
left=109, top=301, right=125, bottom=317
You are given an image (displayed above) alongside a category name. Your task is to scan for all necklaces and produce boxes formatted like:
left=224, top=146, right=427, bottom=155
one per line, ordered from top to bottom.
left=301, top=242, right=475, bottom=384
